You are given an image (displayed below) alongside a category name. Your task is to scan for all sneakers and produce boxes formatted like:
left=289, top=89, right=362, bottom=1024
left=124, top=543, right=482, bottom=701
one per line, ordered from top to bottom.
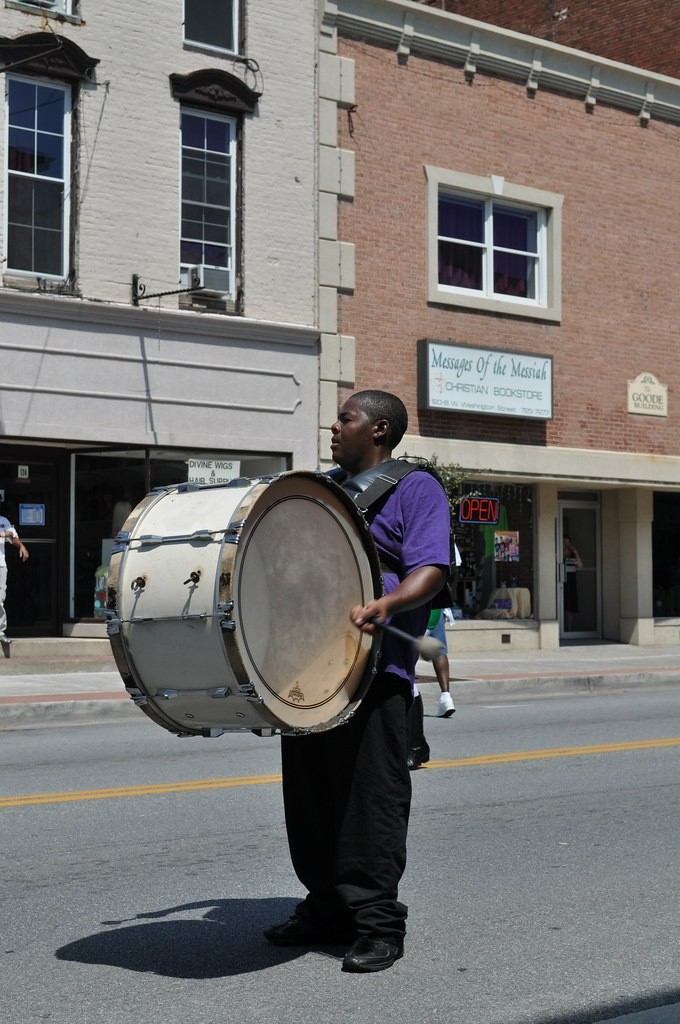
left=434, top=698, right=456, bottom=718
left=262, top=913, right=347, bottom=946
left=342, top=937, right=404, bottom=972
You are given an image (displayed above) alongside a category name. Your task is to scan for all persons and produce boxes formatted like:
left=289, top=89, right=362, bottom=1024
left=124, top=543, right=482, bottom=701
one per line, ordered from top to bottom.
left=262, top=388, right=454, bottom=979
left=0, top=495, right=29, bottom=645
left=561, top=535, right=583, bottom=632
left=495, top=536, right=520, bottom=560
left=415, top=541, right=462, bottom=719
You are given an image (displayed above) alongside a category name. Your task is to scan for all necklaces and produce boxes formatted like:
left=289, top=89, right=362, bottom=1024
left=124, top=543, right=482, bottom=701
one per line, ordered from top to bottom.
left=112, top=500, right=133, bottom=538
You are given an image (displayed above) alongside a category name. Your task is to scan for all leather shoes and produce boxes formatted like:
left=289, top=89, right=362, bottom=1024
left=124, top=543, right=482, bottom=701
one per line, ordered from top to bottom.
left=0, top=635, right=12, bottom=643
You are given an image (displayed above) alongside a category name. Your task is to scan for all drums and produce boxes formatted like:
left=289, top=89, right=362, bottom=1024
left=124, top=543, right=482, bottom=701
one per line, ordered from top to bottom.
left=108, top=469, right=380, bottom=738
